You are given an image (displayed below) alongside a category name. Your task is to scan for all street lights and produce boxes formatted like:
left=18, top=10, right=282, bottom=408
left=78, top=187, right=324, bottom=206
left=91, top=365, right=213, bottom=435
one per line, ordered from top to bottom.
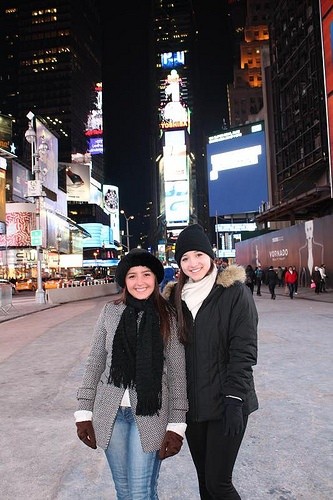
left=24, top=122, right=50, bottom=303
left=119, top=209, right=135, bottom=254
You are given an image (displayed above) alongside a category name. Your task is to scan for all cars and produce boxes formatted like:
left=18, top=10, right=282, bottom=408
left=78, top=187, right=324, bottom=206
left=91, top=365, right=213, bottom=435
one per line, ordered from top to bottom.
left=69, top=273, right=116, bottom=286
left=14, top=277, right=46, bottom=293
left=44, top=277, right=71, bottom=289
left=0, top=279, right=16, bottom=296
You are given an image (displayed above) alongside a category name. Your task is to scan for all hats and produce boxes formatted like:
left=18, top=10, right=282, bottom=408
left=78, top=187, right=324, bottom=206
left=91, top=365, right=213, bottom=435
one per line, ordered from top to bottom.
left=174, top=224, right=214, bottom=268
left=114, top=248, right=165, bottom=288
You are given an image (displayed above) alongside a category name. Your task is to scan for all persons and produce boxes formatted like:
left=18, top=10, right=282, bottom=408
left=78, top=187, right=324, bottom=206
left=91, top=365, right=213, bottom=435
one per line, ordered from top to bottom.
left=298, top=220, right=324, bottom=277
left=162, top=224, right=258, bottom=500
left=74, top=248, right=188, bottom=500
left=217, top=257, right=327, bottom=300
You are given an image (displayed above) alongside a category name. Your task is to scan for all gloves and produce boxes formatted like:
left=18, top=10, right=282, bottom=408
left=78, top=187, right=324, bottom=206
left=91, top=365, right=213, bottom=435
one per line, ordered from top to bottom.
left=223, top=398, right=244, bottom=438
left=75, top=421, right=97, bottom=449
left=157, top=430, right=184, bottom=459
